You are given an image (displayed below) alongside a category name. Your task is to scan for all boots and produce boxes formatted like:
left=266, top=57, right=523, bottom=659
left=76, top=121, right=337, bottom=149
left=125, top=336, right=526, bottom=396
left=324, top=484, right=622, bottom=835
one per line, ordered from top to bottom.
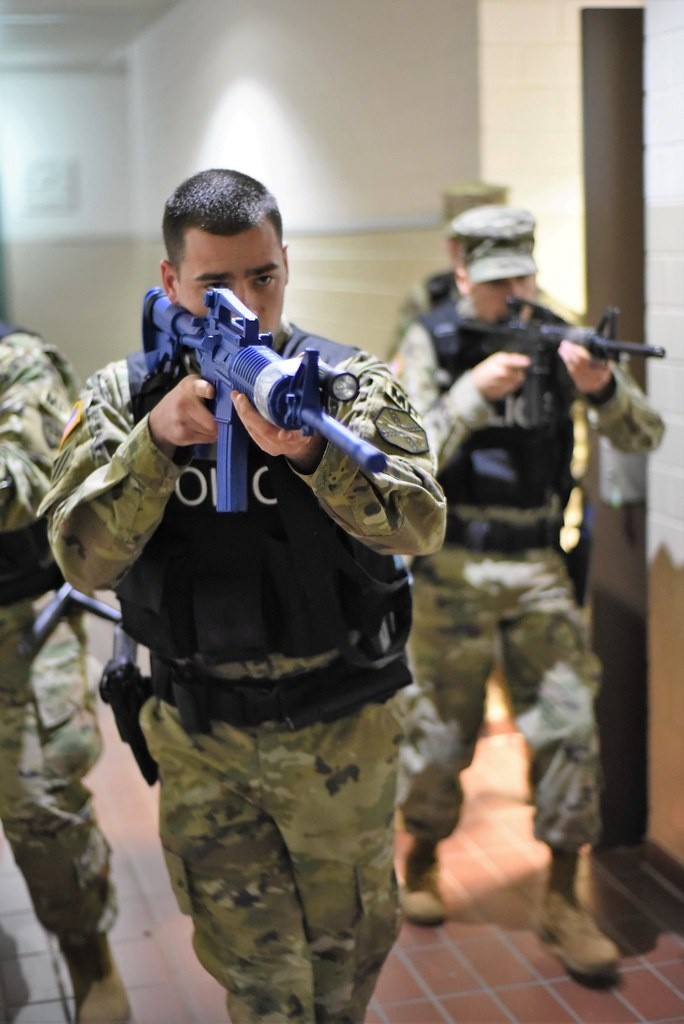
left=399, top=849, right=446, bottom=926
left=535, top=854, right=625, bottom=982
left=61, top=929, right=132, bottom=1024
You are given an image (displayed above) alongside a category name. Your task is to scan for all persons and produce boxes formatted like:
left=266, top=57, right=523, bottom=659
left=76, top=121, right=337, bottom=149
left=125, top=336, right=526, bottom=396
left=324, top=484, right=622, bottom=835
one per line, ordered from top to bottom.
left=36, top=168, right=446, bottom=1024
left=385, top=183, right=666, bottom=980
left=0, top=322, right=132, bottom=1024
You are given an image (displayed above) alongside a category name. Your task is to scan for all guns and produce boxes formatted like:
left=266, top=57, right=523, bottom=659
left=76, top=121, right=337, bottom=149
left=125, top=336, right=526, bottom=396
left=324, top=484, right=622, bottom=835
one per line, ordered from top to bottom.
left=141, top=287, right=389, bottom=513
left=454, top=307, right=666, bottom=427
left=99, top=624, right=160, bottom=787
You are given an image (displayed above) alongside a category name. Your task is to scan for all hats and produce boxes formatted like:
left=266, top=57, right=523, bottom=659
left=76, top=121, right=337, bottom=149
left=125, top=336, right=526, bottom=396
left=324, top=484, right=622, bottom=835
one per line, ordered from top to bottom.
left=439, top=183, right=510, bottom=225
left=446, top=204, right=539, bottom=285
